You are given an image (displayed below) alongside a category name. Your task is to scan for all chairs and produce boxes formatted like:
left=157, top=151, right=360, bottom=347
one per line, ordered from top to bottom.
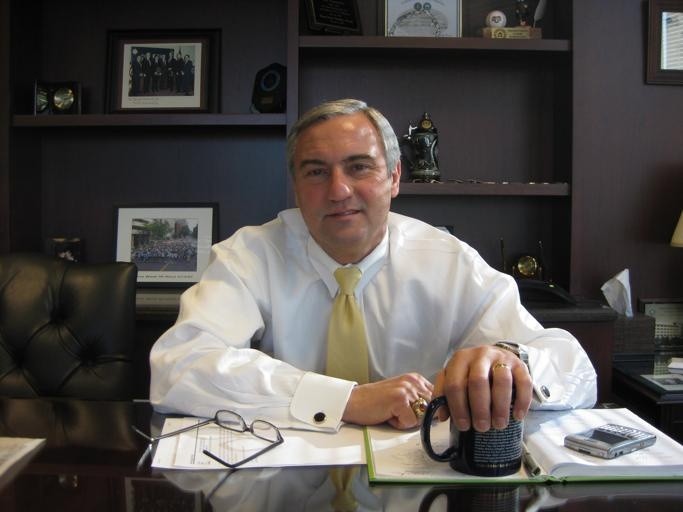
left=0, top=248, right=139, bottom=400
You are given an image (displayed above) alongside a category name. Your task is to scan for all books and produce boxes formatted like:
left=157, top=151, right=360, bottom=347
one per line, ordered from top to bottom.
left=362, top=407, right=683, bottom=483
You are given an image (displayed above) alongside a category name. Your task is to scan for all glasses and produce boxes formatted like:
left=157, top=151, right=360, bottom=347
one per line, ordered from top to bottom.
left=131, top=409, right=285, bottom=468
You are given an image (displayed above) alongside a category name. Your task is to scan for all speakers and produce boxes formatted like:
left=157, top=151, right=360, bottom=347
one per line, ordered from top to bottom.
left=252, top=62, right=286, bottom=113
left=46, top=79, right=88, bottom=114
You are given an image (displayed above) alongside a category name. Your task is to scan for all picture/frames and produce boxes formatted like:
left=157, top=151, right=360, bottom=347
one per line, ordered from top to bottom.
left=384, top=0, right=462, bottom=39
left=645, top=1, right=683, bottom=86
left=103, top=27, right=222, bottom=115
left=109, top=200, right=219, bottom=289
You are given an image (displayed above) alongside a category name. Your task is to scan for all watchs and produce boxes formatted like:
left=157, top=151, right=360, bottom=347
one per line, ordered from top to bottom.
left=494, top=341, right=531, bottom=371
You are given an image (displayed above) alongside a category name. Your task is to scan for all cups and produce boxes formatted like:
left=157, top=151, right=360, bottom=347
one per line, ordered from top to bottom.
left=420, top=378, right=524, bottom=478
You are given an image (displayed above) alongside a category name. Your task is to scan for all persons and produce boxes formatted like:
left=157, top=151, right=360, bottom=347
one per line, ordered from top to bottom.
left=130, top=46, right=194, bottom=97
left=148, top=96, right=600, bottom=434
left=132, top=236, right=195, bottom=263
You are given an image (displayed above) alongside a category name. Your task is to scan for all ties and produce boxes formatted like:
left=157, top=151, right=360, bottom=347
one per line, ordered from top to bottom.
left=326, top=267, right=369, bottom=385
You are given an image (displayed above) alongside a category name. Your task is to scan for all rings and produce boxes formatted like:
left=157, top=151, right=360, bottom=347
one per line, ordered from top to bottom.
left=494, top=363, right=512, bottom=372
left=411, top=398, right=427, bottom=421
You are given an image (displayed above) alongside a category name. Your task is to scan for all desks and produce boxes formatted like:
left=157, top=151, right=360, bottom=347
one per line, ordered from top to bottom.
left=614, top=359, right=682, bottom=443
left=0, top=395, right=682, bottom=511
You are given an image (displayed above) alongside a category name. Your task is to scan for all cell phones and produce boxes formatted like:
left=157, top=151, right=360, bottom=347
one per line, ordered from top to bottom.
left=564, top=423, right=656, bottom=460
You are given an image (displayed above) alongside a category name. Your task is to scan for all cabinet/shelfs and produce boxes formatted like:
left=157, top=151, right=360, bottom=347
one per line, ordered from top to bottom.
left=0, top=1, right=574, bottom=294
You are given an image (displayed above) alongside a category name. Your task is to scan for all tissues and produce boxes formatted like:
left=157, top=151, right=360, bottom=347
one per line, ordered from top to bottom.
left=601, top=269, right=655, bottom=375
